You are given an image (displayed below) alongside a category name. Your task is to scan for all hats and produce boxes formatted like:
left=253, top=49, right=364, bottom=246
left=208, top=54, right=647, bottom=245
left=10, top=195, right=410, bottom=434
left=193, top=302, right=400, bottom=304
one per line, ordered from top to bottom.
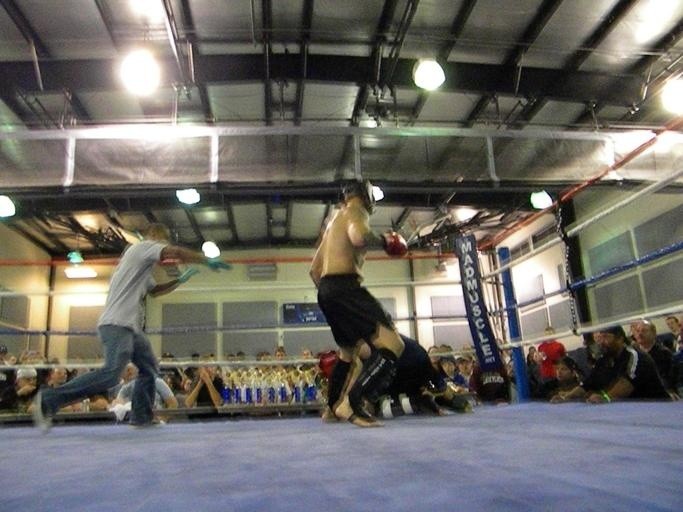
left=343, top=178, right=374, bottom=214
left=552, top=356, right=576, bottom=370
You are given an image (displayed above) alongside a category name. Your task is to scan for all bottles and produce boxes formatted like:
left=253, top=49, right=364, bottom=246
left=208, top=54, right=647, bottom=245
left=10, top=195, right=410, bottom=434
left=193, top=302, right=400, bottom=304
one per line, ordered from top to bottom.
left=223, top=368, right=317, bottom=405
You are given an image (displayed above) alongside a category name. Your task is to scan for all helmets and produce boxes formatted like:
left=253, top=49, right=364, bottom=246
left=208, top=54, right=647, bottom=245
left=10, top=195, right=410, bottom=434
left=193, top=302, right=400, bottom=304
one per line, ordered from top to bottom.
left=319, top=351, right=337, bottom=377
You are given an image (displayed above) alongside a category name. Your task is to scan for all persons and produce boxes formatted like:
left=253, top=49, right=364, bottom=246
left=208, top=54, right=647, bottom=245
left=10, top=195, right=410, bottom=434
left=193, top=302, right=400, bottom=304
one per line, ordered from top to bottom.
left=308, top=178, right=410, bottom=427
left=27, top=222, right=233, bottom=436
left=334, top=316, right=683, bottom=426
left=0, top=344, right=179, bottom=425
left=160, top=346, right=340, bottom=422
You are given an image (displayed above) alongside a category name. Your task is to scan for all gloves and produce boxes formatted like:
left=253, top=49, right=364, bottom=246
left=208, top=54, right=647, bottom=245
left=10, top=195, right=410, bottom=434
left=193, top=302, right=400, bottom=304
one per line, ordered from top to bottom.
left=384, top=230, right=408, bottom=258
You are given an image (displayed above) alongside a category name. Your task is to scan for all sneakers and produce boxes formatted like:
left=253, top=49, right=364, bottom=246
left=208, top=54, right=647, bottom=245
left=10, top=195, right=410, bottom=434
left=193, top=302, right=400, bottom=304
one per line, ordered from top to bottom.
left=34, top=390, right=51, bottom=433
left=130, top=416, right=163, bottom=428
